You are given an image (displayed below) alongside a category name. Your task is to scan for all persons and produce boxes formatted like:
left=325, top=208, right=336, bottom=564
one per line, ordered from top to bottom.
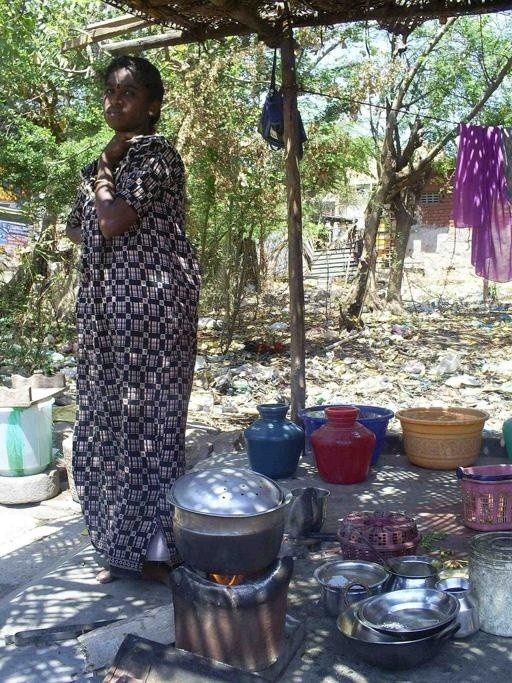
left=63, top=52, right=205, bottom=587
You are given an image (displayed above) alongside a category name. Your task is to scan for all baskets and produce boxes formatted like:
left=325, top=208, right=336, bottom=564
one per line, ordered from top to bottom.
left=454, top=463, right=511, bottom=532
left=338, top=509, right=422, bottom=564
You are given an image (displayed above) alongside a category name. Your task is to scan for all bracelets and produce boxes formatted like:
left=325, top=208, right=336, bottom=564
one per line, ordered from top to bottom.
left=93, top=178, right=108, bottom=186
left=94, top=181, right=115, bottom=194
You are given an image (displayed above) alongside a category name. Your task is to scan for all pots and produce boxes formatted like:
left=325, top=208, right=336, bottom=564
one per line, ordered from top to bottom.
left=160, top=470, right=298, bottom=573
left=386, top=552, right=444, bottom=591
left=315, top=556, right=390, bottom=614
left=332, top=584, right=463, bottom=663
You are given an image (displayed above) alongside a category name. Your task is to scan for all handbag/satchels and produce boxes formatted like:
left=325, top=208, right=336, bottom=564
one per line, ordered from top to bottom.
left=256, top=89, right=309, bottom=150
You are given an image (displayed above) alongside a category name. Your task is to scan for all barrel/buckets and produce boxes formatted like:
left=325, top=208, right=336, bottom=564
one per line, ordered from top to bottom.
left=0, top=399, right=54, bottom=476
left=468, top=530, right=511, bottom=636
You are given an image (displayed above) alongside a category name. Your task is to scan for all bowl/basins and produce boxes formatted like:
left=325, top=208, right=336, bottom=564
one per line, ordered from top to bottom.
left=360, top=593, right=460, bottom=632
left=354, top=609, right=416, bottom=641
